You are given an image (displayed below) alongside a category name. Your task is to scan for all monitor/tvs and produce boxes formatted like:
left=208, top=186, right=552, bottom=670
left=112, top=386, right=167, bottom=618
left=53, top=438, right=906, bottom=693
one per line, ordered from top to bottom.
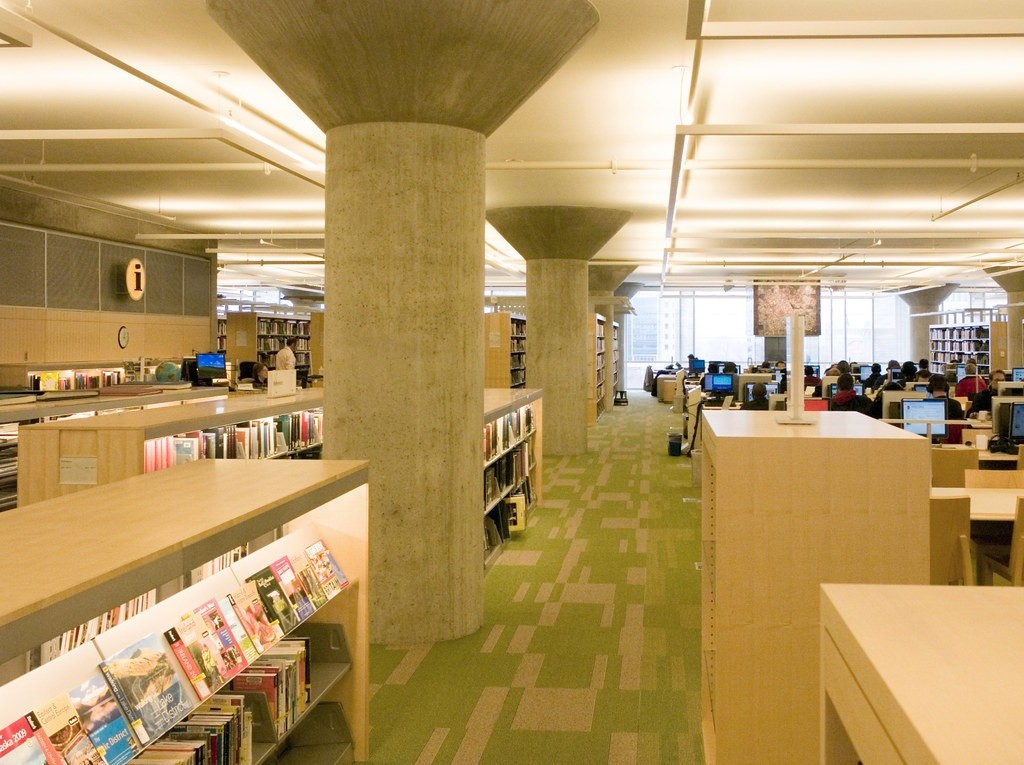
left=196, top=353, right=227, bottom=386
left=181, top=357, right=198, bottom=382
left=689, top=359, right=741, bottom=398
left=294, top=364, right=310, bottom=389
left=745, top=364, right=1024, bottom=444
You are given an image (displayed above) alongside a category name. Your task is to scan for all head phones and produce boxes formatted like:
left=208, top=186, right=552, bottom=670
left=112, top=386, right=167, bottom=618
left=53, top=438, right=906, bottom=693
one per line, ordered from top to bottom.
left=901, top=366, right=917, bottom=374
left=926, top=374, right=950, bottom=394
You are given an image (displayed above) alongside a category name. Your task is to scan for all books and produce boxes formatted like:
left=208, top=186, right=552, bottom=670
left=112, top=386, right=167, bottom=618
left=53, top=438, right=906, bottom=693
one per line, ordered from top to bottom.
left=257, top=317, right=311, bottom=336
left=512, top=320, right=526, bottom=336
left=596, top=324, right=604, bottom=418
left=929, top=327, right=988, bottom=374
left=613, top=328, right=618, bottom=398
left=257, top=335, right=310, bottom=351
left=510, top=339, right=525, bottom=352
left=257, top=353, right=309, bottom=365
left=511, top=354, right=525, bottom=368
left=510, top=370, right=525, bottom=389
left=28, top=371, right=122, bottom=391
left=483, top=405, right=536, bottom=559
left=144, top=407, right=323, bottom=473
left=0, top=434, right=19, bottom=510
left=0, top=519, right=349, bottom=765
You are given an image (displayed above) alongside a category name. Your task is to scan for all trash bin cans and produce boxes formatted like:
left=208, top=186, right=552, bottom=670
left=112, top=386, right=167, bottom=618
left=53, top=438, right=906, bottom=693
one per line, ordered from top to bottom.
left=668, top=433, right=682, bottom=456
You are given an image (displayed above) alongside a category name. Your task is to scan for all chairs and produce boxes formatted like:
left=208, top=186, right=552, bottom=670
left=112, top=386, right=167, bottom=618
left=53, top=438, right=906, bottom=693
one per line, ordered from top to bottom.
left=931, top=448, right=979, bottom=488
left=930, top=492, right=978, bottom=585
left=978, top=497, right=1024, bottom=587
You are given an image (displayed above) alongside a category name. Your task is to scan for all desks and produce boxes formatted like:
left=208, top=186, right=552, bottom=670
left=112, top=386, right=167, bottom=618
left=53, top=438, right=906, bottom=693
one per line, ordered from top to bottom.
left=930, top=487, right=1024, bottom=520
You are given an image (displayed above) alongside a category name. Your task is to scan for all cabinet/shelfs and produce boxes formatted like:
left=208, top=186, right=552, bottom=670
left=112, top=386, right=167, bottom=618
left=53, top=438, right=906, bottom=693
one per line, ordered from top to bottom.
left=0, top=312, right=620, bottom=765
left=929, top=324, right=1009, bottom=372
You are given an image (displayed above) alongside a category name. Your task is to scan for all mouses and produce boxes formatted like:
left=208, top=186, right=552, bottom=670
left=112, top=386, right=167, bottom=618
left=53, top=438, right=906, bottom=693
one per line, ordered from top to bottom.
left=965, top=440, right=972, bottom=446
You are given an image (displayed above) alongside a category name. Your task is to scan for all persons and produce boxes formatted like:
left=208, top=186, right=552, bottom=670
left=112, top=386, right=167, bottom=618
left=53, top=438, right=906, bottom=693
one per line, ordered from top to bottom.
left=275, top=337, right=299, bottom=369
left=688, top=354, right=699, bottom=360
left=775, top=358, right=985, bottom=397
left=700, top=362, right=738, bottom=392
left=762, top=362, right=770, bottom=368
left=253, top=363, right=268, bottom=388
left=966, top=370, right=1007, bottom=419
left=830, top=374, right=873, bottom=413
left=927, top=374, right=964, bottom=420
left=739, top=382, right=769, bottom=411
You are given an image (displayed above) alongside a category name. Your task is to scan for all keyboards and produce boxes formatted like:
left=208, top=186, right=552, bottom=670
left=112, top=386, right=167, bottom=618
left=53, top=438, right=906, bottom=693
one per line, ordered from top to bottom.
left=687, top=378, right=699, bottom=381
left=704, top=402, right=736, bottom=407
